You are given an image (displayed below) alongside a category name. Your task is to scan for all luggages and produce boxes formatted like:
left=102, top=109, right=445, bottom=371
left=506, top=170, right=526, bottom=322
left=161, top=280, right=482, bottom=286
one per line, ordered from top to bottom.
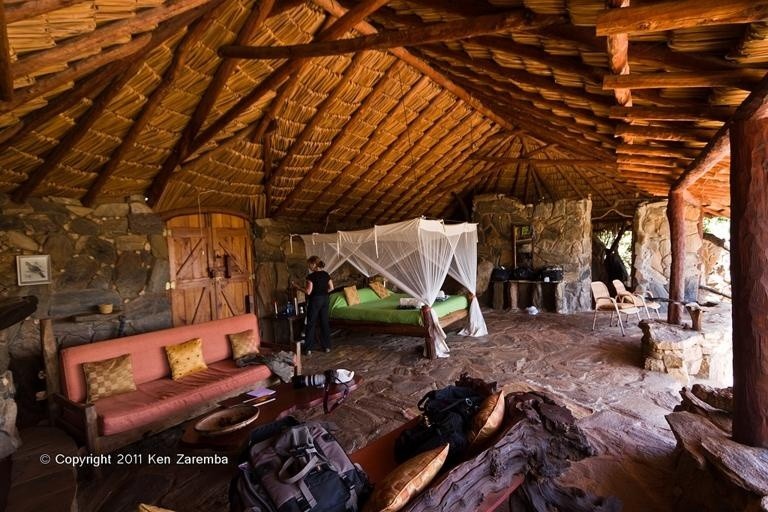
left=539, top=264, right=563, bottom=282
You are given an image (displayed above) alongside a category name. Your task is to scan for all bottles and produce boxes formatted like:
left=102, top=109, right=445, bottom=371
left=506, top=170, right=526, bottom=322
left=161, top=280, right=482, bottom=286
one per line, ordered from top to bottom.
left=287, top=301, right=292, bottom=317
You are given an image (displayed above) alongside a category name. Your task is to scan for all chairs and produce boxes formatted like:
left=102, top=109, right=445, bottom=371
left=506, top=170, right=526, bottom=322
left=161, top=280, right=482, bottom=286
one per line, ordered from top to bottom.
left=591, top=280, right=661, bottom=337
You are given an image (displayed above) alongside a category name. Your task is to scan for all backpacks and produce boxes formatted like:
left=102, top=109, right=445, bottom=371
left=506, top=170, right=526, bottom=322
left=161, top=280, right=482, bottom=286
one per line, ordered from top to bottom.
left=419, top=372, right=498, bottom=418
left=392, top=413, right=465, bottom=466
left=230, top=414, right=372, bottom=512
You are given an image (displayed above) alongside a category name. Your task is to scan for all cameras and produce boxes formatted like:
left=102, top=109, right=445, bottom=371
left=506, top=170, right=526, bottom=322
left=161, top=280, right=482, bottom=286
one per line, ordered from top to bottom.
left=291, top=369, right=339, bottom=389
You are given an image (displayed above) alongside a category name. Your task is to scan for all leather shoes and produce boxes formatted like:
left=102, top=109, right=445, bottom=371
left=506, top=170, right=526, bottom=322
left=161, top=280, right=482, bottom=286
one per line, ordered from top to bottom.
left=325, top=348, right=331, bottom=353
left=306, top=349, right=311, bottom=356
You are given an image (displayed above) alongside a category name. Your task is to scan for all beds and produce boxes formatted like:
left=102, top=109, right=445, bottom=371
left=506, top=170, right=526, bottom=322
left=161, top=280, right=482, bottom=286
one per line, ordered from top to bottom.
left=305, top=286, right=473, bottom=360
left=179, top=376, right=366, bottom=450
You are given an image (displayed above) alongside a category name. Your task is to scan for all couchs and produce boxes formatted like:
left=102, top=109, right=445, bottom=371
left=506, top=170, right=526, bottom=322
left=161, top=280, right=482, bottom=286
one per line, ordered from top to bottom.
left=40, top=295, right=302, bottom=454
left=350, top=390, right=623, bottom=512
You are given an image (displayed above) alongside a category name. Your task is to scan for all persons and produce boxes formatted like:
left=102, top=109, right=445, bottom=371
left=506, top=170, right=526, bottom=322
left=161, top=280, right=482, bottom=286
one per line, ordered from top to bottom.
left=292, top=256, right=335, bottom=356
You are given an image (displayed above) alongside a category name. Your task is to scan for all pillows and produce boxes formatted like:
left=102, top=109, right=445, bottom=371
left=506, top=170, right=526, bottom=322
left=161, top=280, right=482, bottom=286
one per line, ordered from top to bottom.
left=329, top=281, right=393, bottom=307
left=227, top=329, right=260, bottom=360
left=164, top=337, right=208, bottom=381
left=82, top=354, right=137, bottom=404
left=467, top=390, right=505, bottom=453
left=363, top=442, right=450, bottom=512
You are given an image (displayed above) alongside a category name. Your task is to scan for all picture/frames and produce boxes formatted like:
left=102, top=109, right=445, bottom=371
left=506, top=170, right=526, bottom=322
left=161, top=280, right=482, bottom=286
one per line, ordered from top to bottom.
left=15, top=254, right=52, bottom=286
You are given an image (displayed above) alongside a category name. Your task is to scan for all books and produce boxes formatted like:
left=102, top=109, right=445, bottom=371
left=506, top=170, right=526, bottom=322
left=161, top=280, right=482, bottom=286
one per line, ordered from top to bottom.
left=246, top=386, right=276, bottom=397
left=242, top=396, right=276, bottom=407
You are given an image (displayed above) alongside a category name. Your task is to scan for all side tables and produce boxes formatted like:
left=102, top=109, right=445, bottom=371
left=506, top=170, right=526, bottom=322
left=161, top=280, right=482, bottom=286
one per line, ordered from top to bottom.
left=260, top=310, right=306, bottom=344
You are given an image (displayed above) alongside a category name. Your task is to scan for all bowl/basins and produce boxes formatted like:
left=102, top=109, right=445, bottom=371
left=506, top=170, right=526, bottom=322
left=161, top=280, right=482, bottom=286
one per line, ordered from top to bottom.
left=98, top=303, right=113, bottom=314
